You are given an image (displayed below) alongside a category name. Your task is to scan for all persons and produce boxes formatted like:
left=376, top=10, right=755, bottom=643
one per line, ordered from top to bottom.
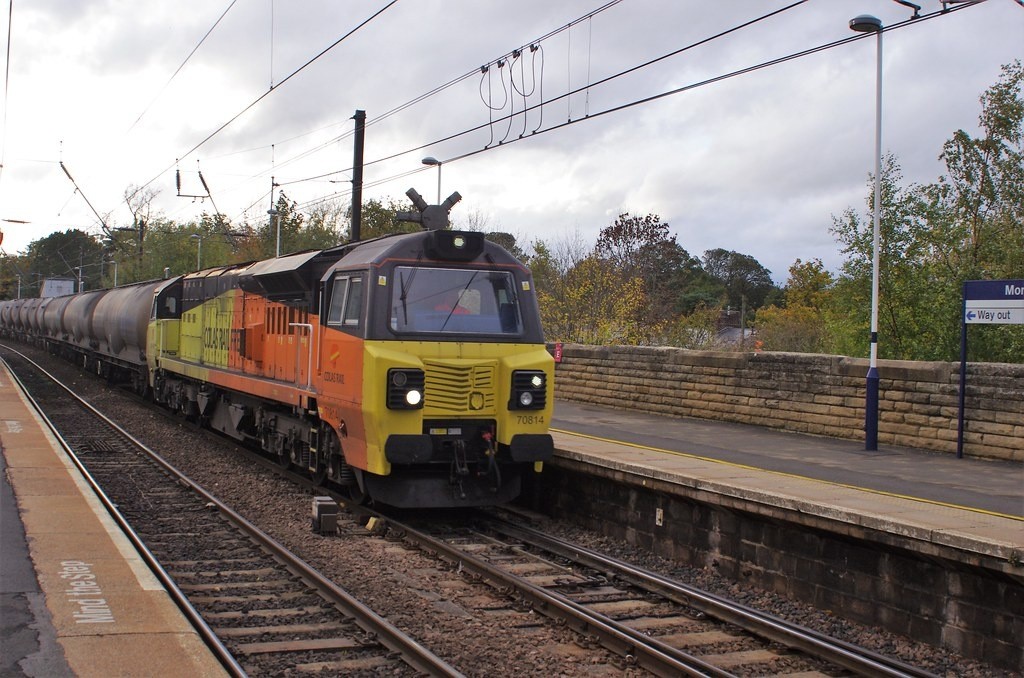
left=434, top=288, right=470, bottom=315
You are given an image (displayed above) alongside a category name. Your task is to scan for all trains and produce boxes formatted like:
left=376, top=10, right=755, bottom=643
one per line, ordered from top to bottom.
left=0, top=109, right=555, bottom=511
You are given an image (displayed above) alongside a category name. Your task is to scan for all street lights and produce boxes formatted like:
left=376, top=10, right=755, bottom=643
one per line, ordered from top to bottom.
left=848, top=14, right=884, bottom=448
left=421, top=157, right=442, bottom=205
left=267, top=209, right=280, bottom=258
left=191, top=234, right=201, bottom=272
left=109, top=261, right=118, bottom=286
left=74, top=267, right=82, bottom=293
left=16, top=274, right=21, bottom=299
